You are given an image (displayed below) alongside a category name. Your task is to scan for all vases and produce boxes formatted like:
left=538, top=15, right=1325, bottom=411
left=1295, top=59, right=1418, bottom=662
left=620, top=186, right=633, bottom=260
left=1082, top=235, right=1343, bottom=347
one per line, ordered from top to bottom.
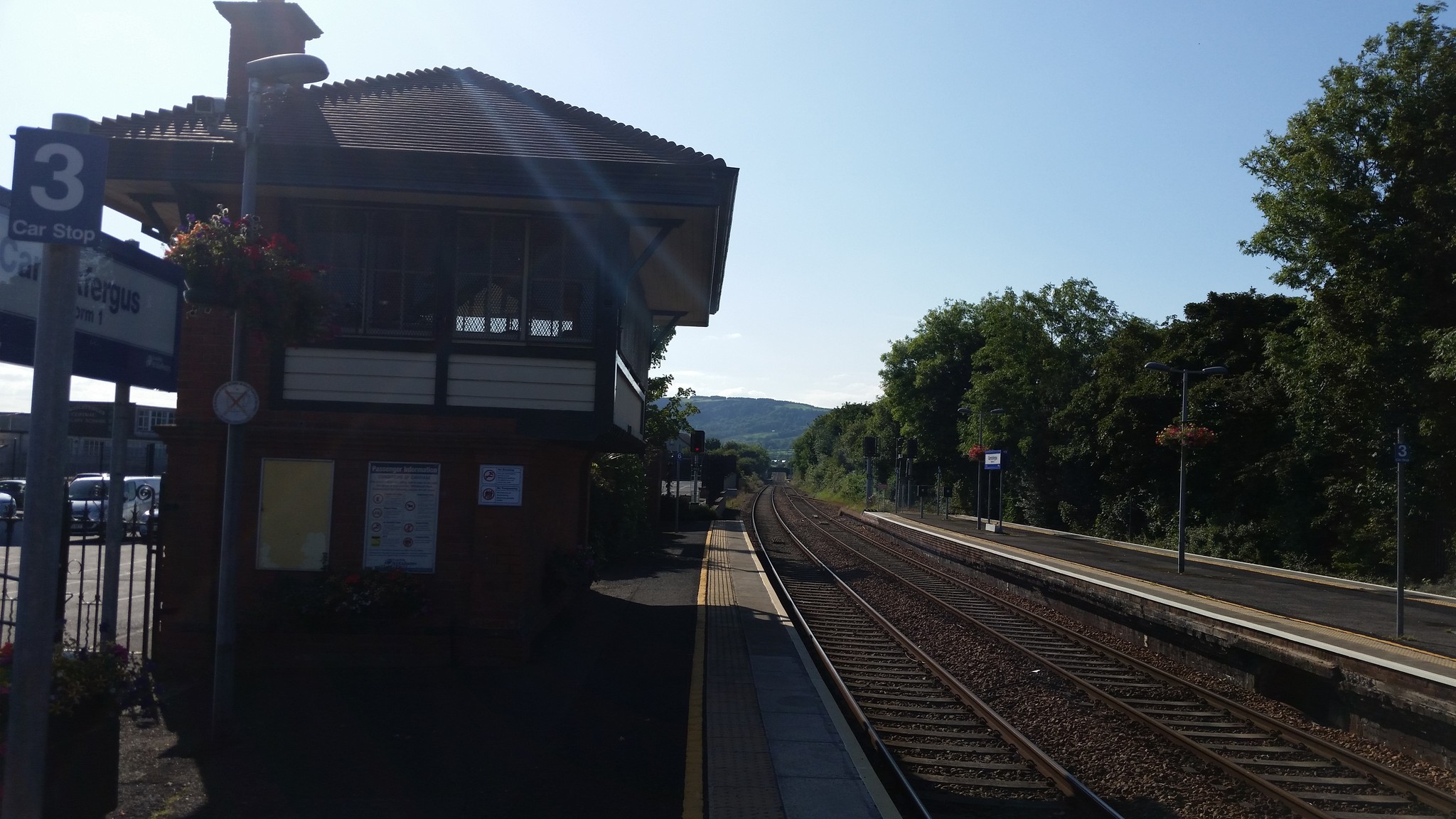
left=40, top=713, right=121, bottom=819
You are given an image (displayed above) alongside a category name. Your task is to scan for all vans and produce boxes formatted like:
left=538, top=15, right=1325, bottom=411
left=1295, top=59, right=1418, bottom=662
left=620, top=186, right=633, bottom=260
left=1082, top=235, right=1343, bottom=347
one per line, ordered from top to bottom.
left=67, top=476, right=162, bottom=543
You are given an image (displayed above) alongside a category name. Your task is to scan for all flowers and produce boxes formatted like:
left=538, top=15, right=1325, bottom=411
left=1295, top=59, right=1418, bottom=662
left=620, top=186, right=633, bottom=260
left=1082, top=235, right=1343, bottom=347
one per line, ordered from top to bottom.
left=166, top=203, right=341, bottom=358
left=1155, top=424, right=1214, bottom=452
left=967, top=444, right=991, bottom=462
left=543, top=542, right=598, bottom=587
left=262, top=549, right=428, bottom=624
left=0, top=617, right=166, bottom=797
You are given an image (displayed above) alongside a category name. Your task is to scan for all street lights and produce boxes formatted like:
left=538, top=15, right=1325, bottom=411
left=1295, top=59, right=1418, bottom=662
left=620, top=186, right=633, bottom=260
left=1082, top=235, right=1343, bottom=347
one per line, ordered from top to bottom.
left=1144, top=361, right=1227, bottom=575
left=957, top=408, right=1004, bottom=530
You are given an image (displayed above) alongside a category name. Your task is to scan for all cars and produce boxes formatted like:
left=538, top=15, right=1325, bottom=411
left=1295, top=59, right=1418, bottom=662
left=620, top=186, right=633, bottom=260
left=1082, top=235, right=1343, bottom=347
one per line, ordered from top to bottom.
left=69, top=473, right=108, bottom=485
left=0, top=480, right=26, bottom=511
left=0, top=492, right=17, bottom=517
left=139, top=508, right=159, bottom=544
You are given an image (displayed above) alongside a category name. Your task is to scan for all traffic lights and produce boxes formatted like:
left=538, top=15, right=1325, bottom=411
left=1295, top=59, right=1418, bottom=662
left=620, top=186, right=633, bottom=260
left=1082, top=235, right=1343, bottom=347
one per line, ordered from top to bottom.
left=690, top=430, right=705, bottom=453
